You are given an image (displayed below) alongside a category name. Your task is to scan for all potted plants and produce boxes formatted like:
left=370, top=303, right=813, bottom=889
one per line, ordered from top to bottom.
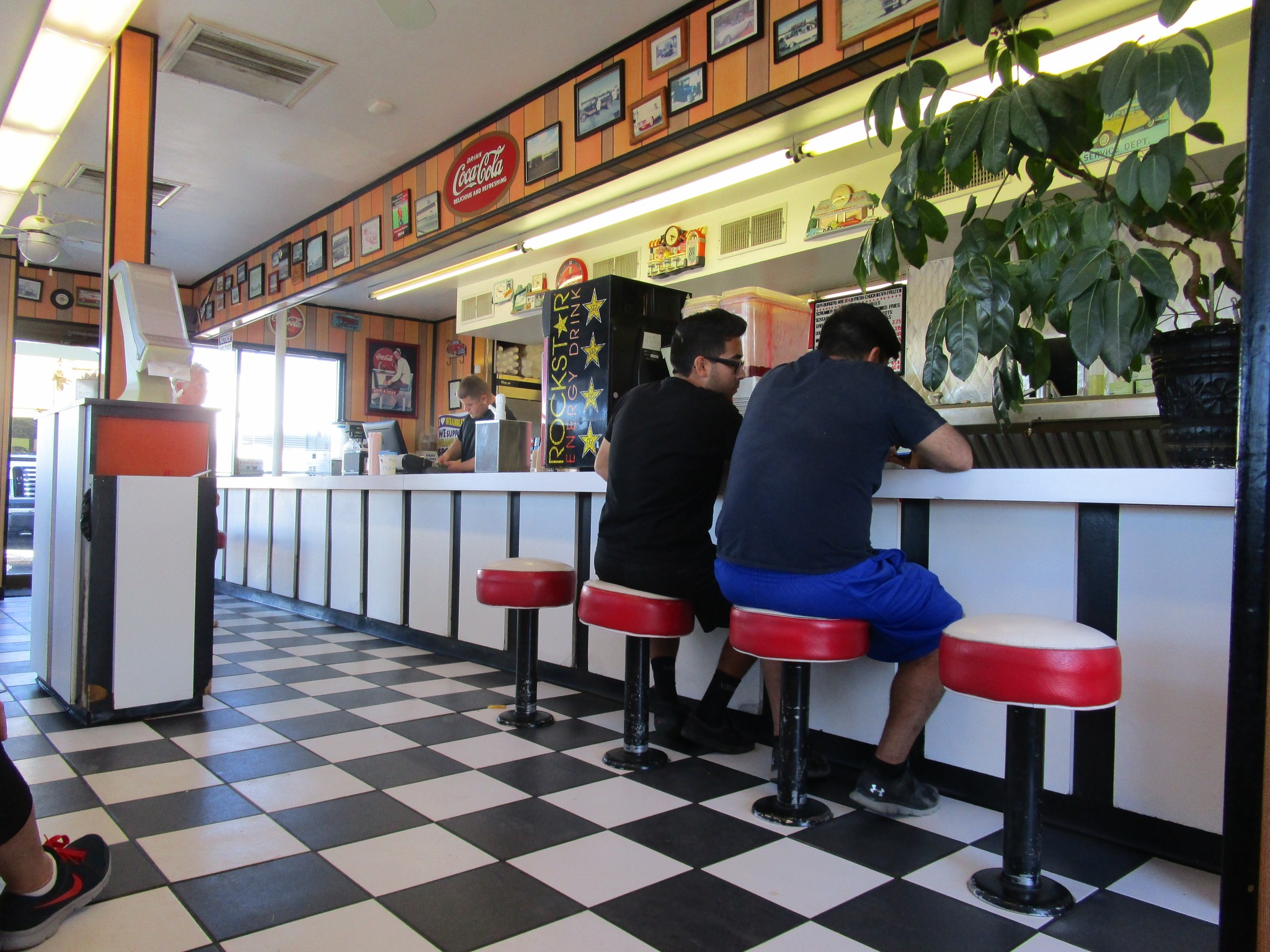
left=848, top=0, right=1245, bottom=471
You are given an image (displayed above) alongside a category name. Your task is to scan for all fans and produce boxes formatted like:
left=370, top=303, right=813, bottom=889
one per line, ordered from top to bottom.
left=0, top=181, right=156, bottom=276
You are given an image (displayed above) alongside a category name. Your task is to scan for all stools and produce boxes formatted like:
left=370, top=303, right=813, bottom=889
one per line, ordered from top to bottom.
left=475, top=557, right=579, bottom=729
left=576, top=577, right=696, bottom=772
left=727, top=603, right=870, bottom=828
left=939, top=611, right=1124, bottom=917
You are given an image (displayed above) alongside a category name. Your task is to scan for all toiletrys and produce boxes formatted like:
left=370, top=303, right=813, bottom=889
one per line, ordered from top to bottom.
left=341, top=429, right=361, bottom=475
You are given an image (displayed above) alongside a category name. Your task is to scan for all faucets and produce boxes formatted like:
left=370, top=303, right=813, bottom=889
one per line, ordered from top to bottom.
left=488, top=394, right=507, bottom=420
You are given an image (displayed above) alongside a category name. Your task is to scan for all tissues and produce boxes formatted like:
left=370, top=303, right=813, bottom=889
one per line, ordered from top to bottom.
left=1131, top=355, right=1154, bottom=380
left=1135, top=378, right=1155, bottom=393
left=1108, top=382, right=1135, bottom=395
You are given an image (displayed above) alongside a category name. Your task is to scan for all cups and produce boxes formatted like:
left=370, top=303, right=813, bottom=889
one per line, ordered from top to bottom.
left=495, top=342, right=542, bottom=380
left=378, top=451, right=397, bottom=475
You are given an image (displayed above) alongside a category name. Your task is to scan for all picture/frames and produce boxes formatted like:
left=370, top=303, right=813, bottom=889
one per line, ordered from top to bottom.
left=200, top=0, right=941, bottom=326
left=17, top=275, right=44, bottom=302
left=75, top=287, right=100, bottom=310
left=365, top=338, right=420, bottom=420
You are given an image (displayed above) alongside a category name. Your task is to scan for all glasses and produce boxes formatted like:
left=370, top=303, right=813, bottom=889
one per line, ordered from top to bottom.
left=694, top=355, right=745, bottom=373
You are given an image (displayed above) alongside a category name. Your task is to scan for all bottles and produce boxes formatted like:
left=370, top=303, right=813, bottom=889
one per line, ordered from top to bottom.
left=342, top=431, right=361, bottom=475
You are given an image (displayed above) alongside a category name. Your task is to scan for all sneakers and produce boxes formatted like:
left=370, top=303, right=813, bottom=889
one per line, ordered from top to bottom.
left=682, top=716, right=754, bottom=754
left=656, top=718, right=687, bottom=747
left=769, top=742, right=832, bottom=779
left=849, top=768, right=942, bottom=813
left=1, top=833, right=111, bottom=951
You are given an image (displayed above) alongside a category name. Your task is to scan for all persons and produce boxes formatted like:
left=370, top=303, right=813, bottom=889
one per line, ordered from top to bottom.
left=713, top=302, right=973, bottom=817
left=593, top=308, right=760, bottom=754
left=372, top=348, right=411, bottom=410
left=172, top=363, right=220, bottom=628
left=436, top=375, right=518, bottom=472
left=0, top=740, right=112, bottom=951
